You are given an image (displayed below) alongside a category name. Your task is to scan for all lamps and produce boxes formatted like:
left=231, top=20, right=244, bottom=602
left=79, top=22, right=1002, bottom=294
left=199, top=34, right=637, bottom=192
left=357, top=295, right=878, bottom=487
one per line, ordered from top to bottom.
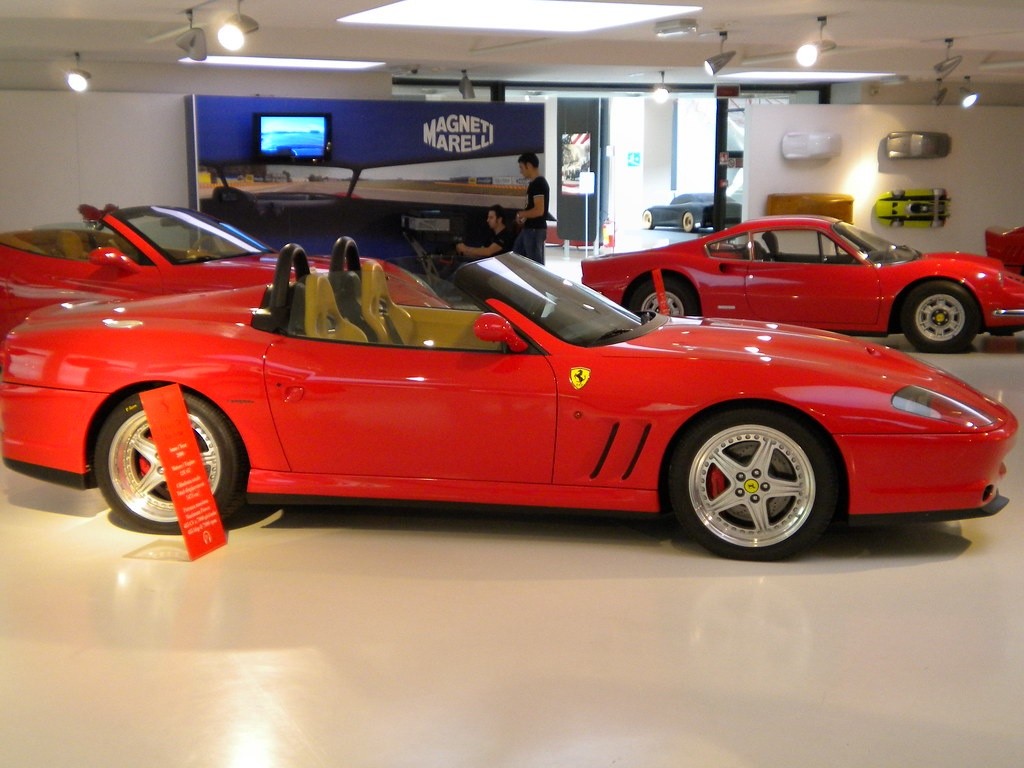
left=217, top=0, right=259, bottom=51
left=175, top=9, right=207, bottom=61
left=795, top=16, right=837, bottom=68
left=704, top=32, right=736, bottom=76
left=934, top=39, right=962, bottom=83
left=67, top=69, right=91, bottom=91
left=459, top=70, right=477, bottom=99
left=652, top=72, right=673, bottom=104
left=958, top=77, right=978, bottom=108
left=932, top=79, right=948, bottom=106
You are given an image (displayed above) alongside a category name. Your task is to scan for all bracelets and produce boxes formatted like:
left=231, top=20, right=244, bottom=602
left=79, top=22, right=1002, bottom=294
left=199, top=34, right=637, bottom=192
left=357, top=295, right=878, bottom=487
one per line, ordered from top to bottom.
left=516, top=212, right=520, bottom=218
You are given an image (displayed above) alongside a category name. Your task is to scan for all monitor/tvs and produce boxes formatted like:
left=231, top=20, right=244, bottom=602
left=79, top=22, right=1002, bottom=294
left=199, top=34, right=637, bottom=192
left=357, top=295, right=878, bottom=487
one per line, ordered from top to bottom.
left=253, top=112, right=332, bottom=163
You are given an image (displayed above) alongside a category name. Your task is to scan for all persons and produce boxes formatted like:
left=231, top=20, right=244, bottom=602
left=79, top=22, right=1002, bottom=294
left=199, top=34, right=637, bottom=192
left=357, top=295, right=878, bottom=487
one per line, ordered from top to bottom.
left=513, top=151, right=549, bottom=264
left=456, top=204, right=521, bottom=257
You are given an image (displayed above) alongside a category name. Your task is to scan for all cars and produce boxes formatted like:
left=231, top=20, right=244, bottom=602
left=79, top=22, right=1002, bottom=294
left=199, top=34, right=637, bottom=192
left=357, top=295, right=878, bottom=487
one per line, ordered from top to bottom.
left=886, top=131, right=952, bottom=159
left=781, top=132, right=840, bottom=159
left=874, top=188, right=952, bottom=229
left=642, top=193, right=741, bottom=233
left=767, top=193, right=854, bottom=225
left=0, top=202, right=454, bottom=351
left=581, top=215, right=1024, bottom=354
left=0, top=238, right=1020, bottom=563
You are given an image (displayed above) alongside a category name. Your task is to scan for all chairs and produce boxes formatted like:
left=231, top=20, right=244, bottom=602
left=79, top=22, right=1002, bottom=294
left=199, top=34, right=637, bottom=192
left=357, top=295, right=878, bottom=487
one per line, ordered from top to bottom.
left=49, top=230, right=89, bottom=261
left=328, top=236, right=425, bottom=347
left=271, top=244, right=369, bottom=343
